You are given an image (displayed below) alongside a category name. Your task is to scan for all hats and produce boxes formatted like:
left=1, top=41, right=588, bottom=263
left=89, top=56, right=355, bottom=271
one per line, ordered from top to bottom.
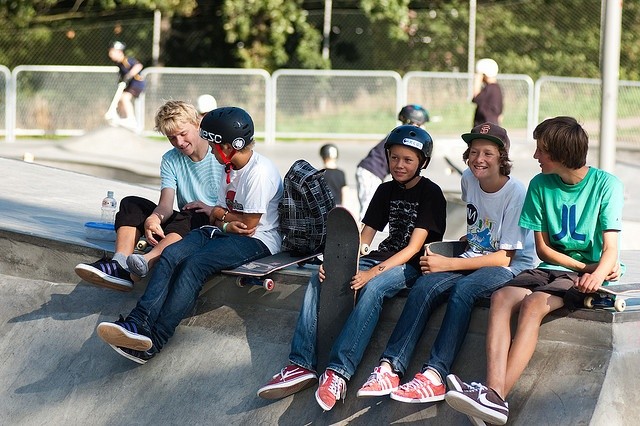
left=461, top=122, right=510, bottom=151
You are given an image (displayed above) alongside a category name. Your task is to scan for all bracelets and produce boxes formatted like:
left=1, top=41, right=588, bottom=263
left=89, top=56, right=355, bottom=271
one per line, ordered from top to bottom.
left=221, top=210, right=229, bottom=220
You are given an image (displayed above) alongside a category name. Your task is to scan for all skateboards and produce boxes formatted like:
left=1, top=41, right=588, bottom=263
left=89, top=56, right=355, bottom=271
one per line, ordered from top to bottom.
left=443, top=157, right=461, bottom=176
left=315, top=207, right=370, bottom=378
left=134, top=235, right=157, bottom=250
left=424, top=238, right=467, bottom=273
left=221, top=247, right=324, bottom=292
left=585, top=282, right=639, bottom=312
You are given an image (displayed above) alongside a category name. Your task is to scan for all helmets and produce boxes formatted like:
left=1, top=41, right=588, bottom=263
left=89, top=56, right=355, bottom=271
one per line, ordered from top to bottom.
left=398, top=105, right=429, bottom=126
left=199, top=107, right=254, bottom=150
left=385, top=125, right=433, bottom=157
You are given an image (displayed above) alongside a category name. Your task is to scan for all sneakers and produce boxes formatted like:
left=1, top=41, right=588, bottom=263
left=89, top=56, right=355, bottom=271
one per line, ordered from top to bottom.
left=74, top=256, right=134, bottom=293
left=97, top=314, right=153, bottom=351
left=108, top=340, right=156, bottom=365
left=257, top=363, right=318, bottom=401
left=357, top=365, right=400, bottom=397
left=315, top=370, right=347, bottom=411
left=390, top=372, right=446, bottom=403
left=447, top=374, right=491, bottom=426
left=444, top=382, right=509, bottom=426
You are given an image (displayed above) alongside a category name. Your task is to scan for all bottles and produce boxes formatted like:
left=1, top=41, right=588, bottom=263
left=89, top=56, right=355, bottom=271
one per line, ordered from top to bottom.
left=100, top=191, right=117, bottom=222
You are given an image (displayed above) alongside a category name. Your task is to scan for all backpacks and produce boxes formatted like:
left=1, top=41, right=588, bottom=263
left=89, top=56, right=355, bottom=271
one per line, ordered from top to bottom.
left=279, top=160, right=335, bottom=256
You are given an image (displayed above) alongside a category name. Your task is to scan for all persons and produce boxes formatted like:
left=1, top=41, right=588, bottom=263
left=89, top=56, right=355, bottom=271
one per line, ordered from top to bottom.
left=106, top=44, right=145, bottom=119
left=442, top=50, right=456, bottom=70
left=472, top=57, right=503, bottom=127
left=76, top=99, right=223, bottom=292
left=355, top=104, right=431, bottom=256
left=96, top=106, right=284, bottom=365
left=444, top=116, right=626, bottom=425
left=356, top=122, right=539, bottom=405
left=257, top=124, right=446, bottom=413
left=321, top=141, right=349, bottom=207
